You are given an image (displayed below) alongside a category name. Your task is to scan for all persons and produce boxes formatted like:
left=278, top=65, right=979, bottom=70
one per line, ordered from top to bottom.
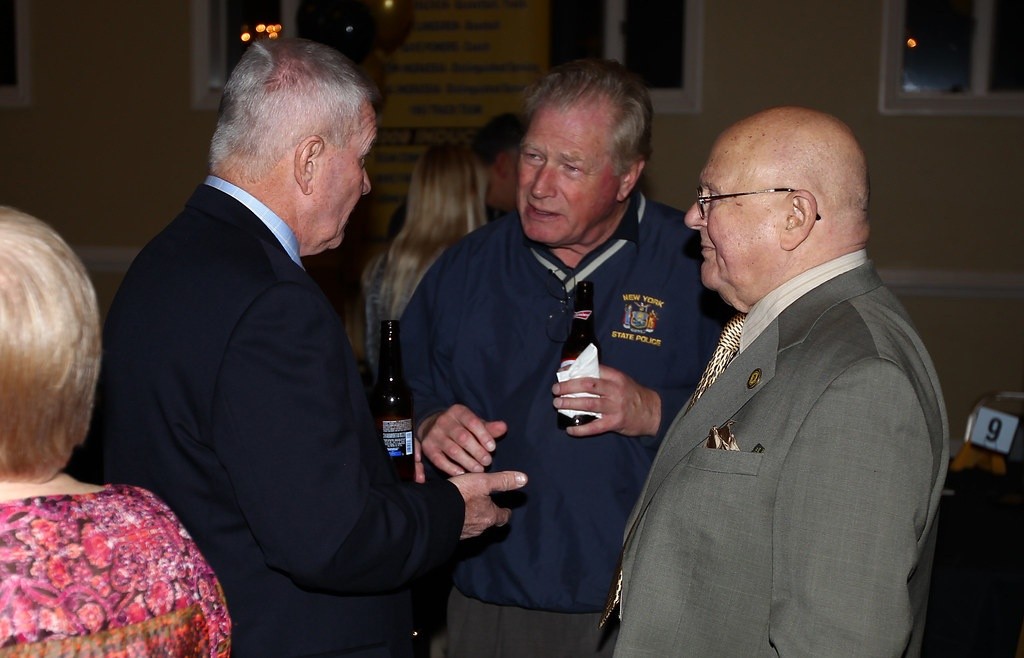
left=99, top=38, right=527, bottom=658
left=400, top=57, right=736, bottom=658
left=595, top=106, right=950, bottom=658
left=360, top=113, right=529, bottom=391
left=0, top=205, right=231, bottom=658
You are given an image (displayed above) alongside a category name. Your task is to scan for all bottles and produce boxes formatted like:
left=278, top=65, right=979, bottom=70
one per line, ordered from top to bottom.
left=557, top=281, right=602, bottom=429
left=368, top=320, right=415, bottom=484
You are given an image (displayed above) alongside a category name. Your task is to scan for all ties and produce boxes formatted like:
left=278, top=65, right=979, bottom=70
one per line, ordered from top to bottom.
left=684, top=313, right=748, bottom=416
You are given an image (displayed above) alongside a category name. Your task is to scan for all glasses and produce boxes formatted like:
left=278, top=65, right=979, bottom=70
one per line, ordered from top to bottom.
left=696, top=186, right=821, bottom=220
